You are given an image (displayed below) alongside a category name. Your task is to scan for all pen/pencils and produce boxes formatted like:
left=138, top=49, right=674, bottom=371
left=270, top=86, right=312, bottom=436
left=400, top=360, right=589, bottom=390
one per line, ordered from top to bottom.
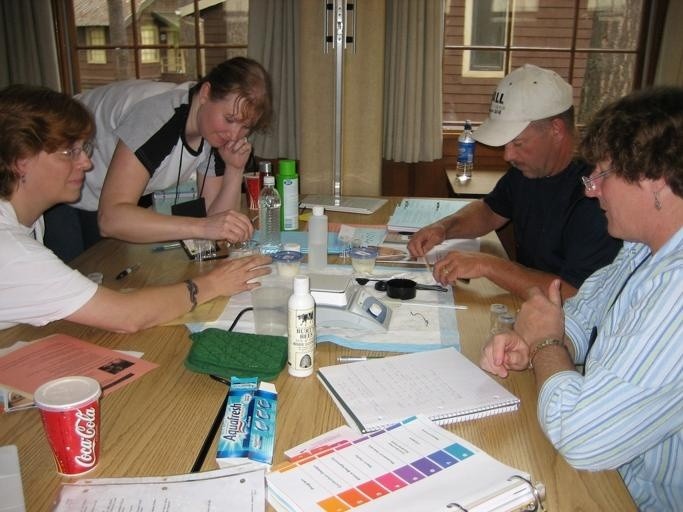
left=153, top=243, right=182, bottom=253
left=116, top=260, right=142, bottom=281
left=338, top=356, right=383, bottom=362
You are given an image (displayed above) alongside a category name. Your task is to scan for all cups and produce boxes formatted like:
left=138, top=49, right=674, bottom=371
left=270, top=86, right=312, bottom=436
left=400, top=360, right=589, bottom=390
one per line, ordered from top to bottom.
left=243, top=172, right=260, bottom=210
left=193, top=238, right=207, bottom=263
left=203, top=239, right=218, bottom=258
left=348, top=248, right=379, bottom=273
left=272, top=250, right=305, bottom=278
left=249, top=287, right=294, bottom=336
left=34, top=375, right=103, bottom=477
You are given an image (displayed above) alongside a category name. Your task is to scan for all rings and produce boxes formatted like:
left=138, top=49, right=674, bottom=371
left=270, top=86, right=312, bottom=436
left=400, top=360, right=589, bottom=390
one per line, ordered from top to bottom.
left=440, top=266, right=449, bottom=275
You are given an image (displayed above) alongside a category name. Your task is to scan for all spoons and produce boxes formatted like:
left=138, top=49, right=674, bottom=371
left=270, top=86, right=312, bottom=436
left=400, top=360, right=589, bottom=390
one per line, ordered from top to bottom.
left=355, top=277, right=382, bottom=286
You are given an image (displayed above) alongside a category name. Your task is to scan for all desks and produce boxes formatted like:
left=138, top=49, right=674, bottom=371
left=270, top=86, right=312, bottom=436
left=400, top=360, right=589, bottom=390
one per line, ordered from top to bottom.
left=442, top=168, right=511, bottom=193
left=0, top=195, right=431, bottom=512
left=197, top=197, right=642, bottom=512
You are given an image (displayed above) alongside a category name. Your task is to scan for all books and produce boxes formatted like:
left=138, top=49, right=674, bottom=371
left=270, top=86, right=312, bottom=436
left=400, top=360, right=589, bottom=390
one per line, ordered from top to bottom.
left=316, top=346, right=521, bottom=438
left=264, top=418, right=542, bottom=512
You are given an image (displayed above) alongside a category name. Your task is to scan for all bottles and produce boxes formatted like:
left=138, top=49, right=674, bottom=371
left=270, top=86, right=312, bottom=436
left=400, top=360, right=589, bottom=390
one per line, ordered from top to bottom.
left=455, top=124, right=476, bottom=183
left=274, top=158, right=300, bottom=231
left=259, top=161, right=274, bottom=190
left=258, top=175, right=282, bottom=254
left=308, top=206, right=328, bottom=274
left=287, top=275, right=317, bottom=378
left=490, top=303, right=508, bottom=339
left=497, top=313, right=514, bottom=334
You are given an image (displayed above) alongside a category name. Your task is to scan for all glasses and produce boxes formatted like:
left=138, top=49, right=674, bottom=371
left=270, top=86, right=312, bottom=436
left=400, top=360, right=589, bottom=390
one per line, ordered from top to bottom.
left=56, top=142, right=92, bottom=160
left=581, top=167, right=615, bottom=192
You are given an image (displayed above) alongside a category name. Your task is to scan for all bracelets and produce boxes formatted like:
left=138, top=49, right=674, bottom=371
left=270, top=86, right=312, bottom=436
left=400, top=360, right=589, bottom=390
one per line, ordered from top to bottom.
left=184, top=279, right=198, bottom=312
left=527, top=338, right=568, bottom=373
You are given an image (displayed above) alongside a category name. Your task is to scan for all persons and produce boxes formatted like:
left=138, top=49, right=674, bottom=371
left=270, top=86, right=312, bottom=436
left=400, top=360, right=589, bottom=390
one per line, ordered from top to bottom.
left=45, top=57, right=271, bottom=265
left=408, top=63, right=624, bottom=306
left=1, top=85, right=273, bottom=337
left=481, top=85, right=683, bottom=512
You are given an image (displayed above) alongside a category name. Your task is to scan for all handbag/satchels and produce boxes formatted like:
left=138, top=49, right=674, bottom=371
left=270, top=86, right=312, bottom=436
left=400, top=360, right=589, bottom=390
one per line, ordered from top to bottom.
left=184, top=327, right=288, bottom=381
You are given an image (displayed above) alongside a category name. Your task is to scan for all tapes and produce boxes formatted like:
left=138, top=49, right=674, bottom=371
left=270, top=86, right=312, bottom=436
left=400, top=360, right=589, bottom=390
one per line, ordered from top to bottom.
left=386, top=279, right=417, bottom=300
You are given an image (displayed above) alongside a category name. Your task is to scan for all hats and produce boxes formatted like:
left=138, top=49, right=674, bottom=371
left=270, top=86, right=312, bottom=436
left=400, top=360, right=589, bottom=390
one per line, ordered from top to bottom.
left=471, top=63, right=573, bottom=146
